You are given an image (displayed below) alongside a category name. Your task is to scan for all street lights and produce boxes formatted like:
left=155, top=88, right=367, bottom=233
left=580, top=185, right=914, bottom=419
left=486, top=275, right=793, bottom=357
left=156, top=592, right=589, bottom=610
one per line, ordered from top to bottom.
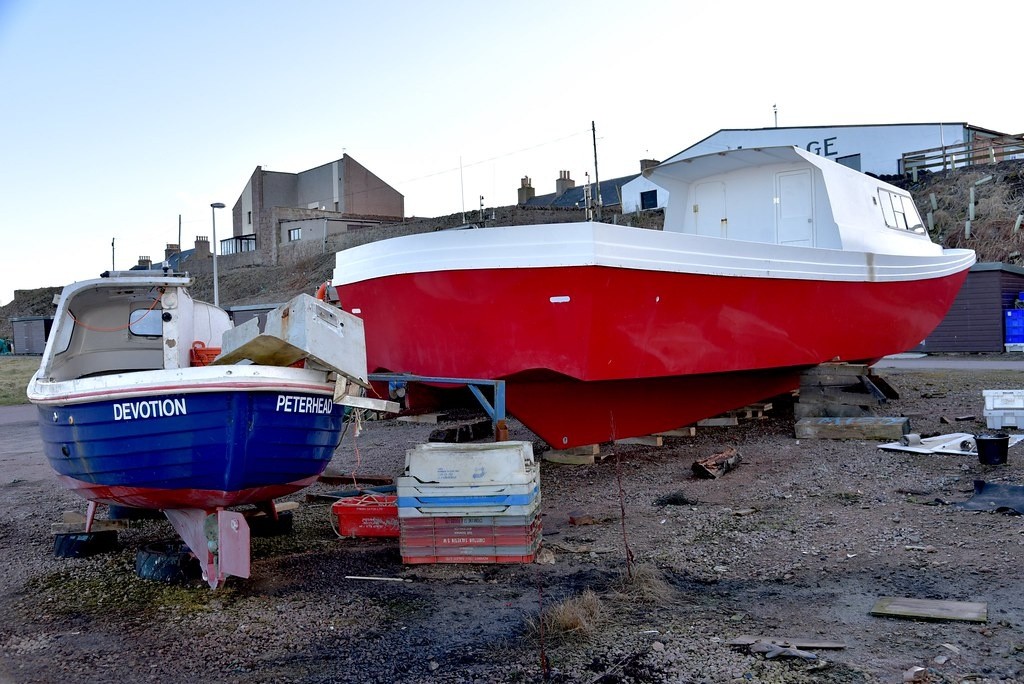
left=210, top=203, right=226, bottom=305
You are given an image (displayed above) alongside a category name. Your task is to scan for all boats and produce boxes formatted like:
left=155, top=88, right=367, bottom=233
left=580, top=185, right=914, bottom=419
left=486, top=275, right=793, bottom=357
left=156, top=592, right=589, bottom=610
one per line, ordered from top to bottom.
left=331, top=143, right=977, bottom=452
left=25, top=275, right=351, bottom=534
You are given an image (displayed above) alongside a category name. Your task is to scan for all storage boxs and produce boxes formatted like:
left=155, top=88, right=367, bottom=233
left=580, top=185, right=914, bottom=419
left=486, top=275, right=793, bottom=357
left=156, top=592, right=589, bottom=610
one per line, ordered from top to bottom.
left=1003, top=290, right=1024, bottom=353
left=221, top=293, right=369, bottom=387
left=982, top=390, right=1024, bottom=430
left=331, top=440, right=543, bottom=565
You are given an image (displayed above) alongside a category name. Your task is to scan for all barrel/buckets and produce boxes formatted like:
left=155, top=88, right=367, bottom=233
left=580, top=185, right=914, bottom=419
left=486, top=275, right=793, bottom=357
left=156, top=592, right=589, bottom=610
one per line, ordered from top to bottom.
left=190, top=341, right=221, bottom=367
left=973, top=432, right=1011, bottom=465
